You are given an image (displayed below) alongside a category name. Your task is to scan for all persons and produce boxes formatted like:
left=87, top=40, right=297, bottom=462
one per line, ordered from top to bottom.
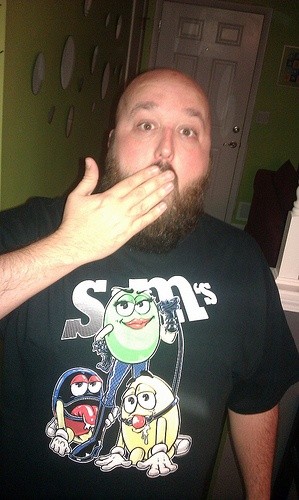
left=0, top=65, right=299, bottom=499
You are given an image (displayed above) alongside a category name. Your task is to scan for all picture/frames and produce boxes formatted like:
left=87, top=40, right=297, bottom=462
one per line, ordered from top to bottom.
left=275, top=44, right=299, bottom=90
